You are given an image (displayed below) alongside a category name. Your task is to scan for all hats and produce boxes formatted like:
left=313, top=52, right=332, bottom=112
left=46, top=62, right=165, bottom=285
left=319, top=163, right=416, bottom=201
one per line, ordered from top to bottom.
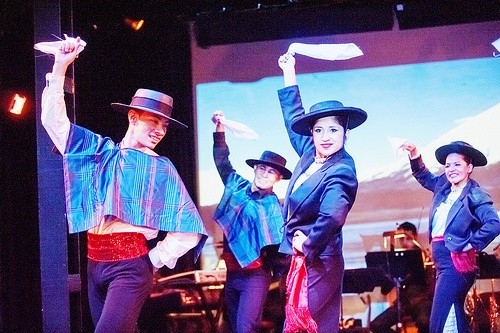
left=111, top=88, right=188, bottom=129
left=291, top=100, right=367, bottom=136
left=435, top=140, right=487, bottom=167
left=246, top=150, right=292, bottom=180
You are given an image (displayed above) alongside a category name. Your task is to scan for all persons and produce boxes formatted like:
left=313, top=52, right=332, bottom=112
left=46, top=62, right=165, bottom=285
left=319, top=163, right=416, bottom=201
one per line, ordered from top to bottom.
left=42, top=36, right=208, bottom=333
left=209, top=52, right=368, bottom=333
left=397, top=139, right=500, bottom=333
left=369, top=222, right=431, bottom=333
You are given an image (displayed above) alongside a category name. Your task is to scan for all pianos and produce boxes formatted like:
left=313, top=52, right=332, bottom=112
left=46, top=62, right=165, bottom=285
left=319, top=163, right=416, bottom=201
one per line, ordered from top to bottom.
left=149, top=268, right=228, bottom=333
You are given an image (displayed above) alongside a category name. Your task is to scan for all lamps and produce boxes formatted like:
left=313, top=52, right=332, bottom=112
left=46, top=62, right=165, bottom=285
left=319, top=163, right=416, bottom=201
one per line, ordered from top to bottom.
left=125, top=19, right=144, bottom=30
